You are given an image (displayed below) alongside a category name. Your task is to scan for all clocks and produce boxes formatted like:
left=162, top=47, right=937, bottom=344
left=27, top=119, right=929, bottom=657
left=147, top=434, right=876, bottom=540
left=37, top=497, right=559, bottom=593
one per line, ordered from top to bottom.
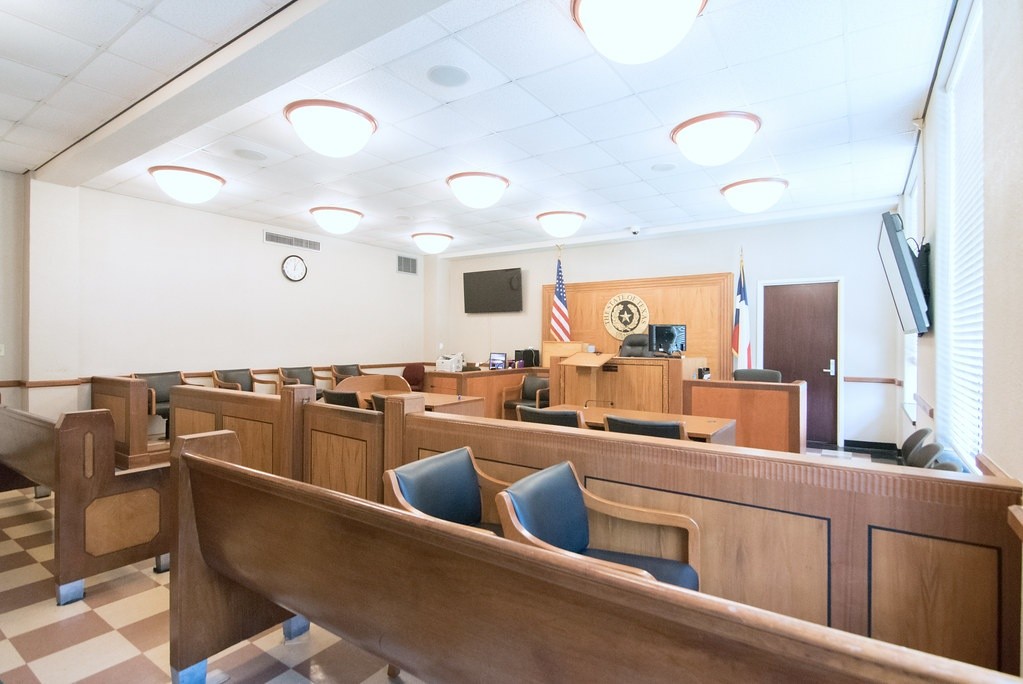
left=281, top=255, right=308, bottom=281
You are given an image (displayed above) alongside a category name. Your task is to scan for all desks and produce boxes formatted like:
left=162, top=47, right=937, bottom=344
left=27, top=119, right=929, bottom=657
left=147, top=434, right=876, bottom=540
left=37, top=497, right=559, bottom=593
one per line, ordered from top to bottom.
left=365, top=390, right=485, bottom=418
left=541, top=404, right=736, bottom=447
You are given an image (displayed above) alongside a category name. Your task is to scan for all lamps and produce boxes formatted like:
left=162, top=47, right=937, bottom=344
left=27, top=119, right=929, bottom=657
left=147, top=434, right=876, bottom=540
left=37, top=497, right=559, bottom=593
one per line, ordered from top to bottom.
left=570, top=0, right=708, bottom=63
left=283, top=100, right=378, bottom=157
left=671, top=110, right=763, bottom=168
left=148, top=166, right=226, bottom=204
left=447, top=171, right=510, bottom=208
left=720, top=178, right=790, bottom=216
left=309, top=207, right=363, bottom=235
left=536, top=210, right=587, bottom=238
left=412, top=233, right=453, bottom=253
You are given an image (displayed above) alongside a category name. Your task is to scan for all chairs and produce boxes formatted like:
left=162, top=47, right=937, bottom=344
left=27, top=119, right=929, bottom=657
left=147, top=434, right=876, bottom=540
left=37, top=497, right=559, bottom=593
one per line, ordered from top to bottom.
left=620, top=334, right=651, bottom=358
left=131, top=363, right=424, bottom=440
left=733, top=368, right=780, bottom=384
left=502, top=375, right=688, bottom=441
left=898, top=426, right=963, bottom=476
left=384, top=446, right=512, bottom=677
left=494, top=461, right=703, bottom=593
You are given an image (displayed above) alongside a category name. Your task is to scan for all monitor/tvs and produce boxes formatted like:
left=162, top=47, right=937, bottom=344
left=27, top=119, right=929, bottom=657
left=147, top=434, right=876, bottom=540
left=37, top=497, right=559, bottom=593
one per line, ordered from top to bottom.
left=879, top=211, right=931, bottom=332
left=464, top=268, right=523, bottom=314
left=648, top=324, right=686, bottom=358
left=489, top=353, right=507, bottom=370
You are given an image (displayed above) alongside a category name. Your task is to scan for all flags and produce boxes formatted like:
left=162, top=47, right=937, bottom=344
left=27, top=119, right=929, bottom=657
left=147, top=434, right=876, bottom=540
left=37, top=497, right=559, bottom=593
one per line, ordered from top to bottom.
left=731, top=256, right=751, bottom=370
left=550, top=259, right=570, bottom=342
